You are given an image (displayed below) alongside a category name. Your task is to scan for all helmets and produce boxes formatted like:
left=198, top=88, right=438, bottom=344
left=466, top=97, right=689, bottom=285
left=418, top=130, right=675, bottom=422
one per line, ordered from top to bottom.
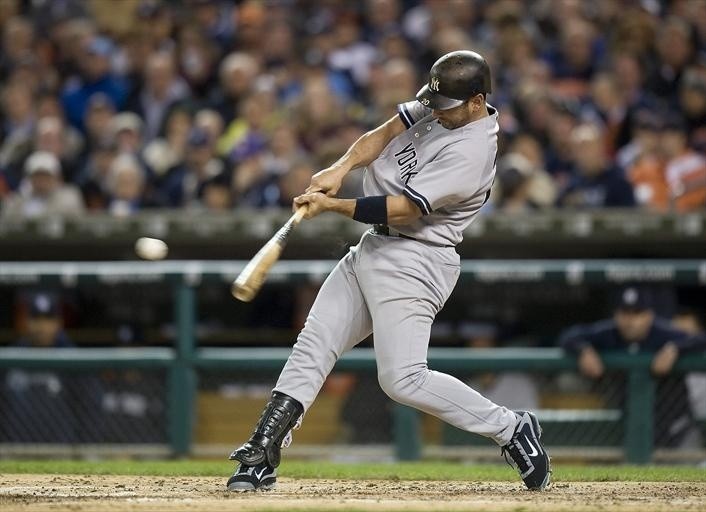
left=415, top=50, right=492, bottom=111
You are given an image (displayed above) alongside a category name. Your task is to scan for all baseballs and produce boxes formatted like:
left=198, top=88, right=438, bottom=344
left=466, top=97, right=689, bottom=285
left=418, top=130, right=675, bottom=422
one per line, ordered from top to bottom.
left=136, top=237, right=169, bottom=261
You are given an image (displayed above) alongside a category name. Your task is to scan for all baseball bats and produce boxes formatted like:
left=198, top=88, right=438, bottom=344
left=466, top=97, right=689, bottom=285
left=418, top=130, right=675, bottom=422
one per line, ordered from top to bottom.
left=231, top=201, right=309, bottom=302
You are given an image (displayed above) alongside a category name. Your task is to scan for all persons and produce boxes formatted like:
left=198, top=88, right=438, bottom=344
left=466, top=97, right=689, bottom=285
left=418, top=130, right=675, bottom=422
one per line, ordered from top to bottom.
left=2, top=1, right=705, bottom=212
left=225, top=49, right=550, bottom=494
left=1, top=288, right=104, bottom=445
left=270, top=288, right=395, bottom=444
left=461, top=289, right=705, bottom=449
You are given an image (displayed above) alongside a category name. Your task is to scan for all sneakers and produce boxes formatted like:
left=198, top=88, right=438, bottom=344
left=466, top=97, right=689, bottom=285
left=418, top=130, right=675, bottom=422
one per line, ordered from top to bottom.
left=501, top=409, right=553, bottom=492
left=226, top=458, right=277, bottom=493
